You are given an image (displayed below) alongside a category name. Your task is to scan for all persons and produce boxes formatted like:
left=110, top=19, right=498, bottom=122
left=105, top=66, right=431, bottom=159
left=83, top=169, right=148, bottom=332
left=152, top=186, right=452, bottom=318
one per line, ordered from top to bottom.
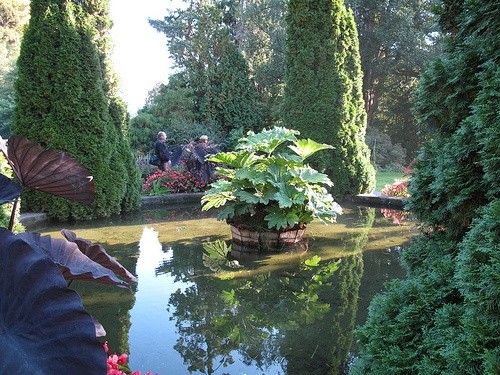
left=156, top=131, right=173, bottom=171
left=196, top=135, right=211, bottom=149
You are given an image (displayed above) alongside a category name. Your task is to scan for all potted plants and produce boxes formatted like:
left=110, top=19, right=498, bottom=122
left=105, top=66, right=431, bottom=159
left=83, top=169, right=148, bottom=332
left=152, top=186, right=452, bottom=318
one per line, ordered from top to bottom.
left=198, top=126, right=343, bottom=251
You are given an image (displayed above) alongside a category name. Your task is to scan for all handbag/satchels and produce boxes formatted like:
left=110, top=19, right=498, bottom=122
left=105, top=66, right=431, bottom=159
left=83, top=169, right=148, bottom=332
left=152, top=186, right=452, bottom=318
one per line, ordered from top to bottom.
left=148, top=153, right=161, bottom=165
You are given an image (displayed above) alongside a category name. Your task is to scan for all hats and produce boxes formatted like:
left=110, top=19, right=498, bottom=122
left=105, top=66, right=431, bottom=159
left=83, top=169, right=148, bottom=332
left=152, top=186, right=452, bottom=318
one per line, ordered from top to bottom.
left=199, top=135, right=208, bottom=140
